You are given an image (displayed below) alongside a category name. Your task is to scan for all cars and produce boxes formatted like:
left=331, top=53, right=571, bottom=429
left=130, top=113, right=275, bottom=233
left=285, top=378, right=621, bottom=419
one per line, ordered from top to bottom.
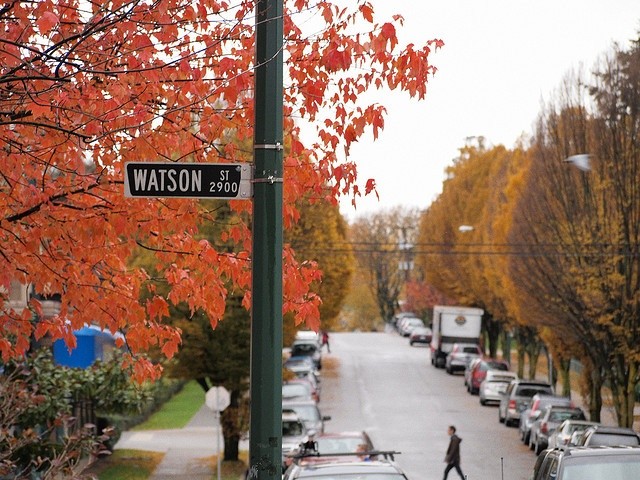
left=399, top=318, right=424, bottom=336
left=410, top=328, right=432, bottom=345
left=293, top=331, right=319, bottom=353
left=446, top=343, right=483, bottom=374
left=289, top=344, right=321, bottom=369
left=287, top=356, right=318, bottom=371
left=468, top=361, right=508, bottom=395
left=294, top=371, right=320, bottom=384
left=480, top=371, right=518, bottom=405
left=288, top=379, right=319, bottom=400
left=281, top=383, right=316, bottom=403
left=520, top=393, right=576, bottom=439
left=282, top=403, right=330, bottom=433
left=524, top=406, right=586, bottom=455
left=548, top=419, right=601, bottom=449
left=286, top=432, right=378, bottom=466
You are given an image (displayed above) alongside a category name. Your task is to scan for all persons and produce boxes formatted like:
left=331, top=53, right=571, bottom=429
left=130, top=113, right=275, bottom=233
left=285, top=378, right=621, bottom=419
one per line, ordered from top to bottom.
left=319, top=331, right=330, bottom=352
left=442, top=426, right=465, bottom=479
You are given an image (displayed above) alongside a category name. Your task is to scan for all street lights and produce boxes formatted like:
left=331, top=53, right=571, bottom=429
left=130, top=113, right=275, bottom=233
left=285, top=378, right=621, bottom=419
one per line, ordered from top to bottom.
left=393, top=225, right=414, bottom=280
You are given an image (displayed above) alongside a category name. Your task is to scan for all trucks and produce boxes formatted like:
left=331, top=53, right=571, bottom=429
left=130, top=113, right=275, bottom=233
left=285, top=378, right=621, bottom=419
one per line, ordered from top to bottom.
left=431, top=307, right=484, bottom=368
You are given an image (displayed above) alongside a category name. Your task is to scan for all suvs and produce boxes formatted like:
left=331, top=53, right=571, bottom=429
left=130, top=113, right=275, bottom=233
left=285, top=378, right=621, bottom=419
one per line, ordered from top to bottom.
left=498, top=380, right=551, bottom=427
left=282, top=409, right=315, bottom=468
left=577, top=425, right=640, bottom=446
left=534, top=447, right=640, bottom=480
left=283, top=451, right=408, bottom=480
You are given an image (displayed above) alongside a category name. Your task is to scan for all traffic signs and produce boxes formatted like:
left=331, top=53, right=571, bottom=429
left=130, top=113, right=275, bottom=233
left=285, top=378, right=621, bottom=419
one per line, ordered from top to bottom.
left=124, top=162, right=251, bottom=199
